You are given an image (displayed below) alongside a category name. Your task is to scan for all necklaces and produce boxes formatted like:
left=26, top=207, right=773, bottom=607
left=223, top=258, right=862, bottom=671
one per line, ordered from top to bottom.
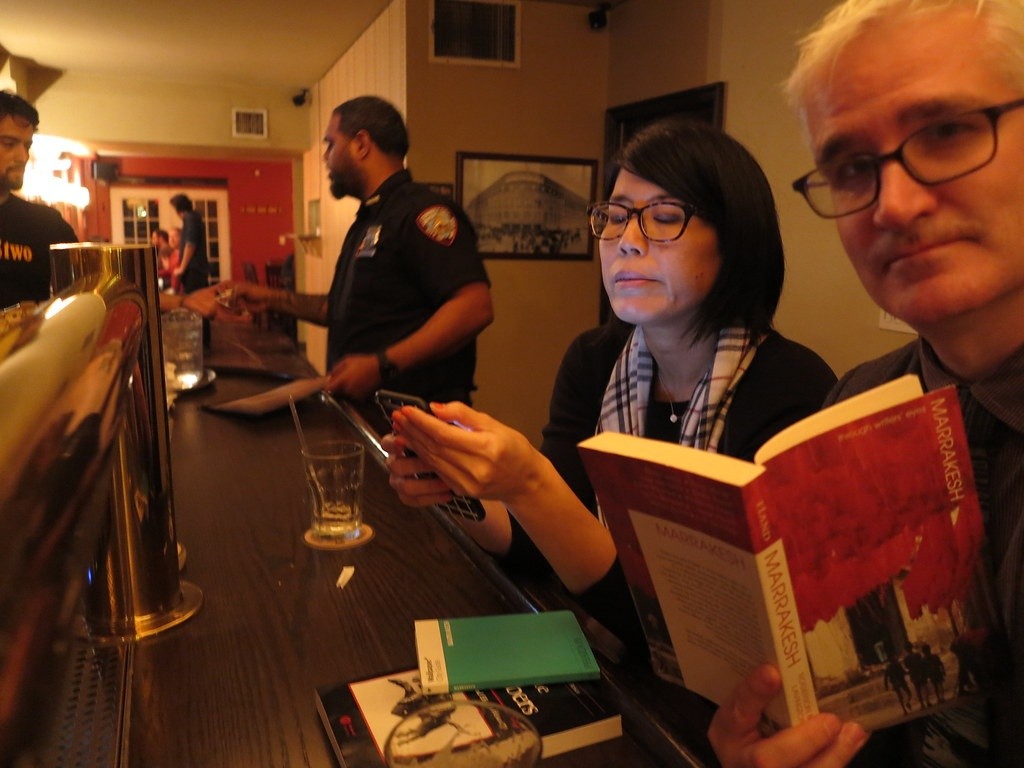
left=657, top=374, right=685, bottom=422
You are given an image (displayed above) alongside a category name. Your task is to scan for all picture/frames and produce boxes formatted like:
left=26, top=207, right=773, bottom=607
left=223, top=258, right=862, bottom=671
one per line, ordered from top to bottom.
left=427, top=0, right=522, bottom=69
left=601, top=82, right=726, bottom=190
left=231, top=107, right=267, bottom=136
left=451, top=150, right=601, bottom=260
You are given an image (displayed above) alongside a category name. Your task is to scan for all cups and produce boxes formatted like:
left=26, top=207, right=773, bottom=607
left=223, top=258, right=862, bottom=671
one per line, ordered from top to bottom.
left=160, top=307, right=203, bottom=383
left=301, top=440, right=364, bottom=540
left=384, top=700, right=543, bottom=768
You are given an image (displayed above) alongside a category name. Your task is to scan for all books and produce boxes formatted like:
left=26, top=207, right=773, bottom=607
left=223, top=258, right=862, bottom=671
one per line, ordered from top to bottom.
left=572, top=374, right=993, bottom=736
left=315, top=614, right=625, bottom=767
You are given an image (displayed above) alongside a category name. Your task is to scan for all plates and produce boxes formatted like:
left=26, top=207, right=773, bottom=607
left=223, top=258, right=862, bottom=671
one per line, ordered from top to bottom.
left=177, top=368, right=216, bottom=391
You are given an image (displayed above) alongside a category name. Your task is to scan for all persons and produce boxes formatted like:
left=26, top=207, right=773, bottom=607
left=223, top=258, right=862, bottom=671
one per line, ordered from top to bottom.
left=704, top=0, right=1024, bottom=767
left=0, top=90, right=253, bottom=328
left=220, top=96, right=492, bottom=428
left=381, top=117, right=839, bottom=768
left=170, top=193, right=213, bottom=356
left=478, top=224, right=584, bottom=256
left=151, top=227, right=184, bottom=295
left=902, top=642, right=932, bottom=711
left=920, top=643, right=947, bottom=704
left=884, top=652, right=913, bottom=716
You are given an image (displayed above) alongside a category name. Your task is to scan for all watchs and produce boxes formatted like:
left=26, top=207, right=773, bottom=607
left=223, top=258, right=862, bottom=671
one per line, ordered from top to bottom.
left=376, top=349, right=398, bottom=381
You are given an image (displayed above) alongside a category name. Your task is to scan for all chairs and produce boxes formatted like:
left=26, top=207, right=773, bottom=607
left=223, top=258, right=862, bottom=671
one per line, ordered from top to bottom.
left=239, top=253, right=295, bottom=340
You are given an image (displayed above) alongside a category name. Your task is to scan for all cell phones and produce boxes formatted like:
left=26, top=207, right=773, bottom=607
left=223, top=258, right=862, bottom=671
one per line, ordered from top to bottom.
left=376, top=390, right=486, bottom=523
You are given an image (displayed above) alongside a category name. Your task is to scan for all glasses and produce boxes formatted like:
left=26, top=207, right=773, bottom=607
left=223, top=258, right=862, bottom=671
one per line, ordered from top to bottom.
left=791, top=99, right=1024, bottom=217
left=585, top=199, right=699, bottom=243
